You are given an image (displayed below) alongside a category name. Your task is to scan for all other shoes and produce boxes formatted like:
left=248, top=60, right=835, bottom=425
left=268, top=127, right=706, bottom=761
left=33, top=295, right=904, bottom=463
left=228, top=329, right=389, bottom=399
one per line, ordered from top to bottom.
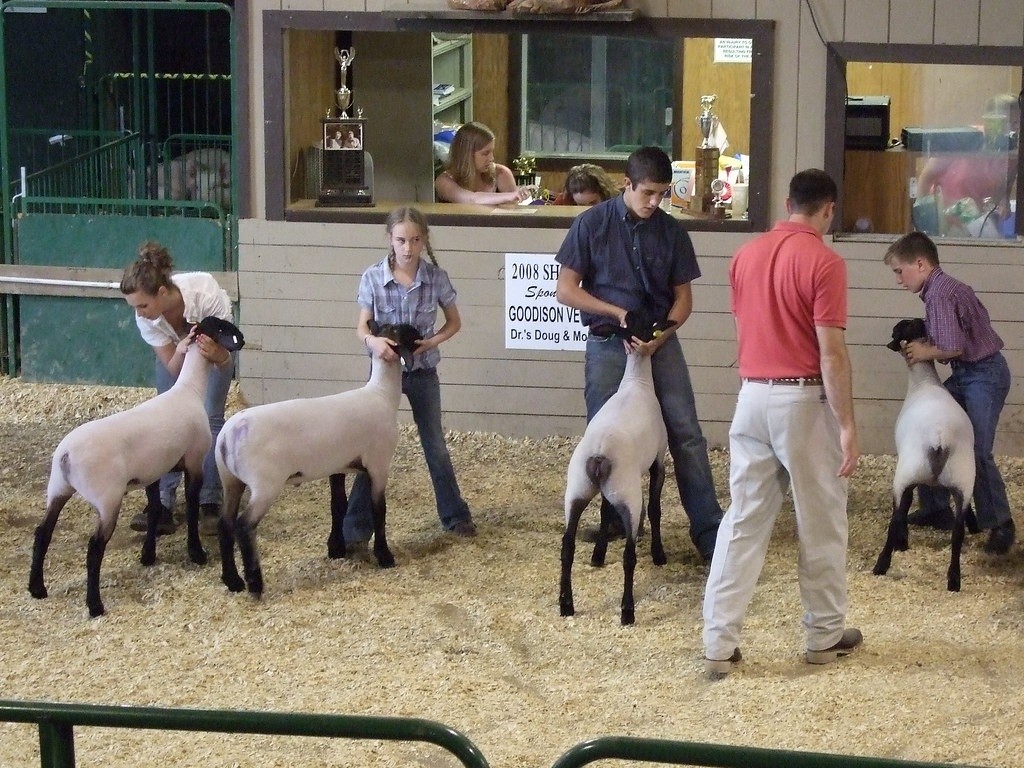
left=198, top=504, right=221, bottom=535
left=130, top=505, right=175, bottom=535
left=908, top=509, right=958, bottom=529
left=446, top=520, right=479, bottom=539
left=985, top=520, right=1019, bottom=554
left=581, top=521, right=645, bottom=542
left=346, top=541, right=370, bottom=562
left=806, top=628, right=864, bottom=664
left=704, top=648, right=742, bottom=674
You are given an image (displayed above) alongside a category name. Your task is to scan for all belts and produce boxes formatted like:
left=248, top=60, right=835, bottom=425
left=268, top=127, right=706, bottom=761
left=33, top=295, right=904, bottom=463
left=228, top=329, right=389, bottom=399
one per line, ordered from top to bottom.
left=747, top=374, right=824, bottom=387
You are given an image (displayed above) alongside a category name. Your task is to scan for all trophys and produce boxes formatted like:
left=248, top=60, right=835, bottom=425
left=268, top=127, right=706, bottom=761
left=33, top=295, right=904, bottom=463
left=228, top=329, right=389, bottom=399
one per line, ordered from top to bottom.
left=320, top=46, right=369, bottom=119
left=695, top=96, right=720, bottom=150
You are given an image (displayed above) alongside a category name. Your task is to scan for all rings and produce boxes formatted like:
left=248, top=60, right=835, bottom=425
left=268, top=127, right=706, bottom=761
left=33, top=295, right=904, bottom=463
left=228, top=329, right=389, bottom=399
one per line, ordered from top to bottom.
left=521, top=193, right=525, bottom=196
left=908, top=354, right=910, bottom=358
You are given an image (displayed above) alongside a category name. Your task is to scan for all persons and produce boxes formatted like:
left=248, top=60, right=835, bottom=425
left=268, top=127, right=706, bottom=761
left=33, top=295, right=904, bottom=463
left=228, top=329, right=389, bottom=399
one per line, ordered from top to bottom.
left=916, top=93, right=1020, bottom=237
left=435, top=121, right=531, bottom=205
left=329, top=131, right=342, bottom=149
left=344, top=131, right=360, bottom=148
left=554, top=147, right=726, bottom=564
left=552, top=163, right=622, bottom=206
left=702, top=168, right=863, bottom=673
left=344, top=207, right=476, bottom=561
left=884, top=232, right=1017, bottom=557
left=121, top=242, right=233, bottom=536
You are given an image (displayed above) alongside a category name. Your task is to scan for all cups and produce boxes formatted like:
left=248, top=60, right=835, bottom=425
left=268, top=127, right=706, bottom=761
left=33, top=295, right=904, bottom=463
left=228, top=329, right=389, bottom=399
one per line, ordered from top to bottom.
left=982, top=115, right=1006, bottom=150
left=731, top=184, right=748, bottom=219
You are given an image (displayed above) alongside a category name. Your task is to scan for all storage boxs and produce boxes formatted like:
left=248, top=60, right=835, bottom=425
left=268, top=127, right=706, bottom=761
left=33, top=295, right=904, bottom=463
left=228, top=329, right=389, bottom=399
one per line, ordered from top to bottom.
left=672, top=161, right=698, bottom=207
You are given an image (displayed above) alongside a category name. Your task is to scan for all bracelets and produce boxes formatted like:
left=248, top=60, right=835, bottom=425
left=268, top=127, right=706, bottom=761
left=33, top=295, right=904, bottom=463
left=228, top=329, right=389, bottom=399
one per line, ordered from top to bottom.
left=364, top=335, right=371, bottom=346
left=214, top=351, right=231, bottom=369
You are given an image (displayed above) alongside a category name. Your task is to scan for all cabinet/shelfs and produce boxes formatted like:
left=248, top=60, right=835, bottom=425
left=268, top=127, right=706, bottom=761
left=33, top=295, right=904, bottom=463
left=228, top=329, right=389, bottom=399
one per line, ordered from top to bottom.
left=352, top=31, right=473, bottom=203
left=843, top=148, right=1019, bottom=234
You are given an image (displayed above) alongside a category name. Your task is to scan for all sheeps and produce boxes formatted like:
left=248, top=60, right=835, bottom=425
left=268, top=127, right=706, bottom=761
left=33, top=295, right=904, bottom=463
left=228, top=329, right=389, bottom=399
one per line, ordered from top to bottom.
left=556, top=310, right=680, bottom=624
left=871, top=314, right=975, bottom=593
left=213, top=316, right=427, bottom=596
left=27, top=317, right=248, bottom=620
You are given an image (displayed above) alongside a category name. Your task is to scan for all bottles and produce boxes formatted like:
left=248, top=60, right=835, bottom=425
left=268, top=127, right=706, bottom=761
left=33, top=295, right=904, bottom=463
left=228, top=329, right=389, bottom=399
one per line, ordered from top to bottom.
left=659, top=183, right=672, bottom=214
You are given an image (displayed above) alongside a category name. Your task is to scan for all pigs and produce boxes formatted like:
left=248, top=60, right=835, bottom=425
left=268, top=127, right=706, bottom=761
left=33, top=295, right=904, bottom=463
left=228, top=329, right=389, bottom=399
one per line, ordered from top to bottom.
left=124, top=148, right=231, bottom=208
